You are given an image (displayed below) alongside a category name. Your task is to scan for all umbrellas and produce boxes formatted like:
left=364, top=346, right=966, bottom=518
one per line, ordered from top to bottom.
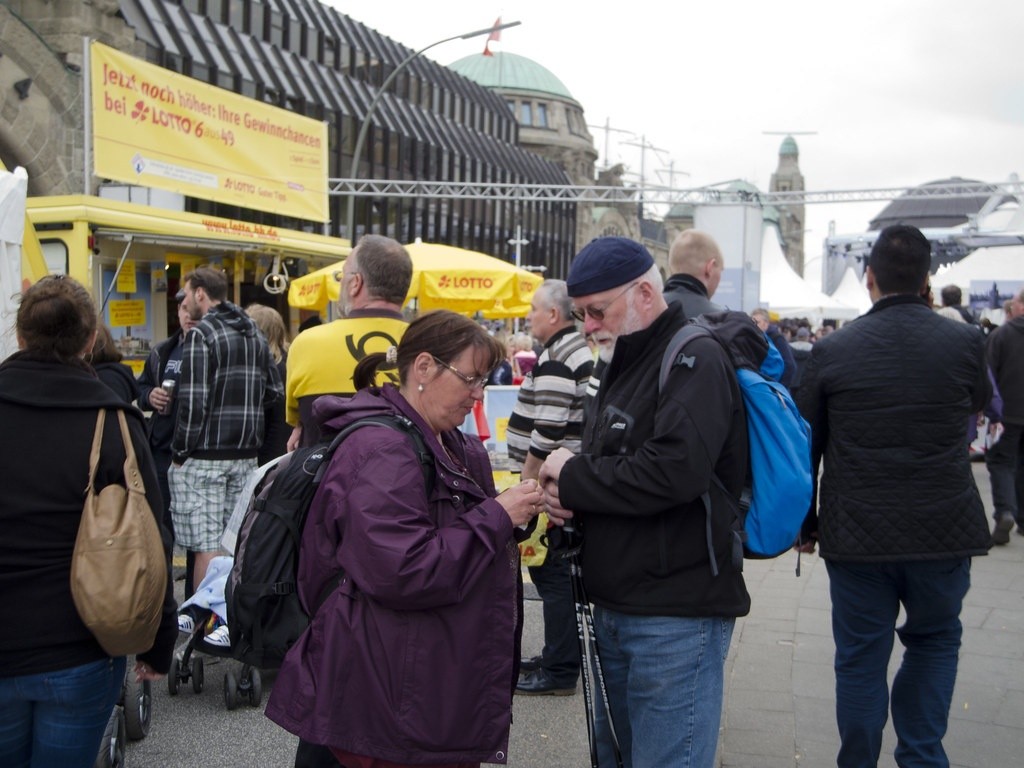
left=288, top=237, right=544, bottom=324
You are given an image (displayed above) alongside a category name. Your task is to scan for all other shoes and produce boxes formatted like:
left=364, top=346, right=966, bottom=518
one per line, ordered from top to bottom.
left=992, top=511, right=1014, bottom=544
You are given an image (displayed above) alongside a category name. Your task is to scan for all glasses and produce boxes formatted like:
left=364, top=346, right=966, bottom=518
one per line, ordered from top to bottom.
left=333, top=270, right=361, bottom=282
left=571, top=282, right=644, bottom=322
left=431, top=357, right=488, bottom=390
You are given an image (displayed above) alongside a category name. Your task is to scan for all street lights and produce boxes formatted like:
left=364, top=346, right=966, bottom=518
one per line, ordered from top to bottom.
left=346, top=19, right=523, bottom=244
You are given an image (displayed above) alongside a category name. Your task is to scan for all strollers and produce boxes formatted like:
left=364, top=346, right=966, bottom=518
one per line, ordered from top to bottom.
left=166, top=451, right=295, bottom=712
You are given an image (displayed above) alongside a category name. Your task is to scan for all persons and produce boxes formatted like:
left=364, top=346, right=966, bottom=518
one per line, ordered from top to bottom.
left=792, top=226, right=995, bottom=768
left=79, top=228, right=1023, bottom=694
left=538, top=236, right=751, bottom=768
left=0, top=274, right=168, bottom=768
left=258, top=309, right=544, bottom=768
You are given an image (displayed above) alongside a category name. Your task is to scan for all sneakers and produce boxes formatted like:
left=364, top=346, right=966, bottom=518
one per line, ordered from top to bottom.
left=178, top=614, right=195, bottom=633
left=203, top=625, right=231, bottom=646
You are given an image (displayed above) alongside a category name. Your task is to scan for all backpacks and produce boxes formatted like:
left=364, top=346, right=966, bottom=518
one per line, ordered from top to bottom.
left=659, top=309, right=815, bottom=560
left=224, top=416, right=433, bottom=665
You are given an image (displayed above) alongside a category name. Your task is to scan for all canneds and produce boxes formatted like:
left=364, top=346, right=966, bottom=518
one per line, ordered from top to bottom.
left=158, top=379, right=176, bottom=415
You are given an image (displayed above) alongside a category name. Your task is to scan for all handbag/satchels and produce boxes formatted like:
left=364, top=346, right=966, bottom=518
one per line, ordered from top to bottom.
left=71, top=409, right=166, bottom=658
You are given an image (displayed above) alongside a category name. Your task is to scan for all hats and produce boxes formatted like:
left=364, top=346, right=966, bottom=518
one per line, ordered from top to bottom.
left=567, top=237, right=656, bottom=297
left=175, top=286, right=185, bottom=302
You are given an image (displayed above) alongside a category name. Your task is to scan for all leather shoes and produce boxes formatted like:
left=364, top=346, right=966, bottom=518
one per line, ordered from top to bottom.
left=514, top=655, right=577, bottom=697
